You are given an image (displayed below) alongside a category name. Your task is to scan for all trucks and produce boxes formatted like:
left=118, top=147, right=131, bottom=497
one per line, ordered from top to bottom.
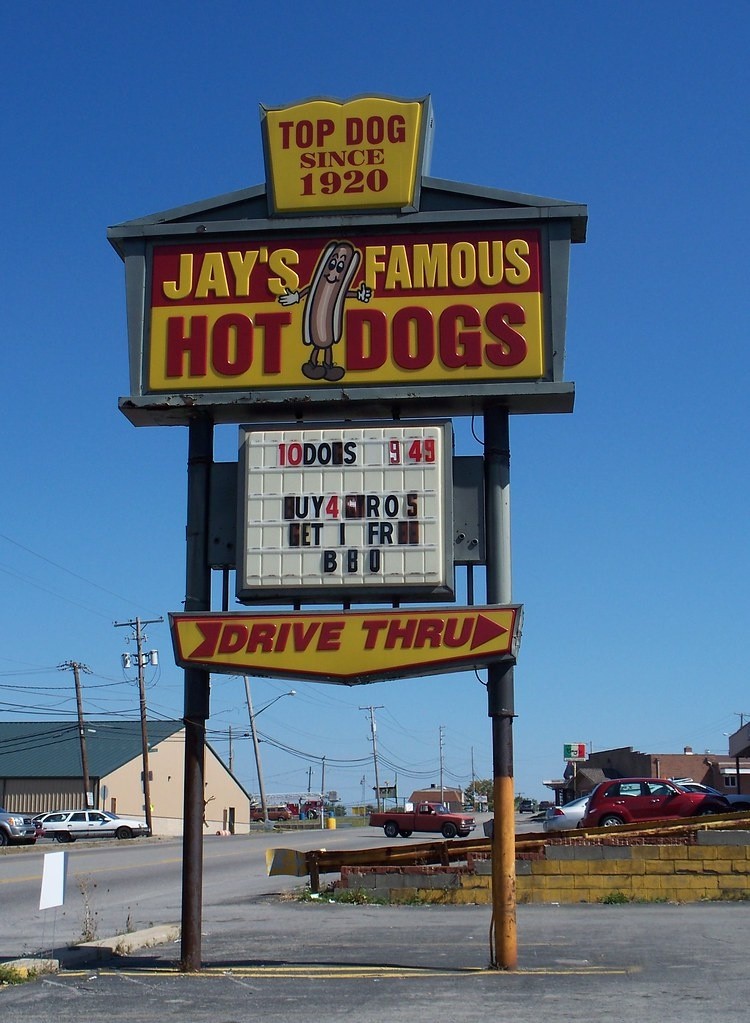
left=285, top=799, right=322, bottom=820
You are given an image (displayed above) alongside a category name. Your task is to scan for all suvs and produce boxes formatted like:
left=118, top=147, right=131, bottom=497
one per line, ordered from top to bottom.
left=519, top=799, right=535, bottom=813
left=539, top=800, right=551, bottom=811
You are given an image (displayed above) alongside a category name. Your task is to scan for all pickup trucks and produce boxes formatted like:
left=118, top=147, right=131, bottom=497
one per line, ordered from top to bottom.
left=369, top=802, right=477, bottom=838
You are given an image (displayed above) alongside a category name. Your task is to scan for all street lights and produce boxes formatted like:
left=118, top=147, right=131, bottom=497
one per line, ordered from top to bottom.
left=122, top=648, right=159, bottom=838
left=250, top=690, right=297, bottom=828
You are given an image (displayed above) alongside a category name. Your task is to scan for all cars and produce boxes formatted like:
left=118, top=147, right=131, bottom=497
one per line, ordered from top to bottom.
left=583, top=777, right=731, bottom=828
left=619, top=781, right=750, bottom=813
left=543, top=785, right=659, bottom=833
left=0, top=807, right=38, bottom=846
left=40, top=808, right=148, bottom=843
left=29, top=811, right=65, bottom=838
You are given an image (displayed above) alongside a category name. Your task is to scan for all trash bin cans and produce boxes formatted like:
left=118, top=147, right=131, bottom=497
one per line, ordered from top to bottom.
left=327, top=817, right=336, bottom=829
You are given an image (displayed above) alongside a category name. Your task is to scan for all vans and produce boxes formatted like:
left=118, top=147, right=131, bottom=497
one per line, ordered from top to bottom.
left=253, top=805, right=293, bottom=823
